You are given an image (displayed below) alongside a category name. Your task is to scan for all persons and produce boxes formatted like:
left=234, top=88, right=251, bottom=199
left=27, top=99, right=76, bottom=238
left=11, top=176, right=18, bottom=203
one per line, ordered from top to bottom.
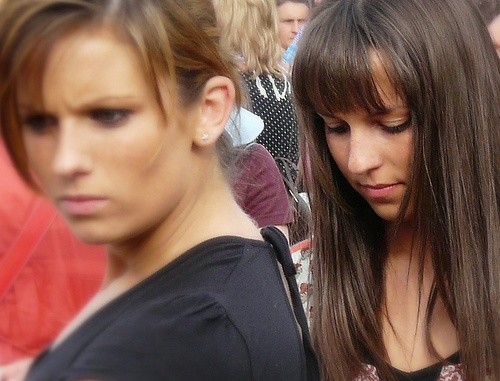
left=0, top=0, right=308, bottom=381
left=217, top=0, right=312, bottom=245
left=480, top=1, right=500, bottom=58
left=295, top=2, right=499, bottom=381
